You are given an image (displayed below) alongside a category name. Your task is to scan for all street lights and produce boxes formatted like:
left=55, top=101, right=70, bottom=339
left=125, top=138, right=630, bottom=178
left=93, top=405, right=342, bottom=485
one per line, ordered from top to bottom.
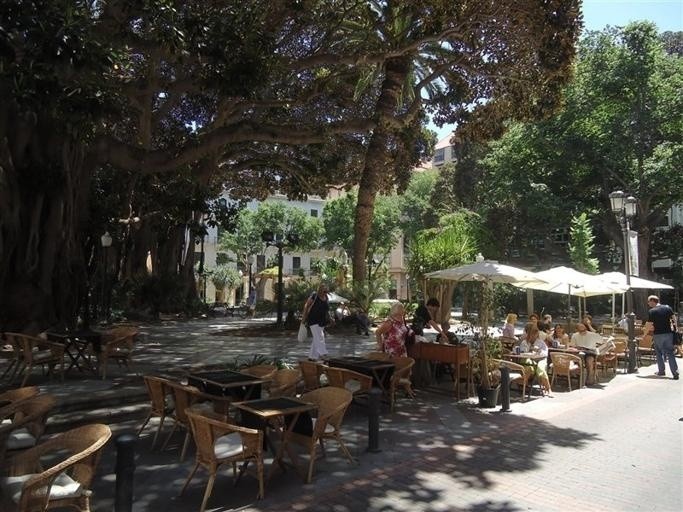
left=608, top=191, right=637, bottom=373
left=98, top=231, right=113, bottom=381
left=261, top=232, right=295, bottom=330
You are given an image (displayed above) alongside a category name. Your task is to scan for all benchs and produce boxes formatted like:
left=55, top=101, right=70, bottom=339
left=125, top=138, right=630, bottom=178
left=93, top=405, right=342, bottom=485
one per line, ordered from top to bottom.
left=224, top=302, right=252, bottom=319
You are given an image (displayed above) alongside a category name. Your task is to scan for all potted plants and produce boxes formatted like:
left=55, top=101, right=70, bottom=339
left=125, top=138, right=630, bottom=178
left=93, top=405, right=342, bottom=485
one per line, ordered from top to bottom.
left=455, top=275, right=527, bottom=408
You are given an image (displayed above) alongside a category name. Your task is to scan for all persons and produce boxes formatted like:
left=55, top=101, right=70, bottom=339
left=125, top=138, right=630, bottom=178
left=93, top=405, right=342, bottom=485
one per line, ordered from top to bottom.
left=301, top=283, right=329, bottom=362
left=248, top=286, right=258, bottom=318
left=644, top=295, right=679, bottom=380
left=410, top=298, right=447, bottom=340
left=335, top=299, right=377, bottom=337
left=376, top=302, right=416, bottom=397
left=502, top=312, right=569, bottom=398
left=583, top=316, right=598, bottom=333
left=436, top=321, right=458, bottom=345
left=570, top=323, right=602, bottom=386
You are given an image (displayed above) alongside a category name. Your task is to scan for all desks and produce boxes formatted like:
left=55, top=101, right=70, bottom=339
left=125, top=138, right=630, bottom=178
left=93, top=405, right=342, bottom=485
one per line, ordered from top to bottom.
left=414, top=341, right=470, bottom=402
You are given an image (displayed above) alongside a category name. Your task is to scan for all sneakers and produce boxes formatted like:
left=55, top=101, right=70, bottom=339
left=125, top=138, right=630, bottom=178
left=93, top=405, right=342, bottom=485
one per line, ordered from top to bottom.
left=321, top=355, right=328, bottom=360
left=307, top=357, right=318, bottom=362
left=654, top=372, right=665, bottom=376
left=673, top=374, right=679, bottom=380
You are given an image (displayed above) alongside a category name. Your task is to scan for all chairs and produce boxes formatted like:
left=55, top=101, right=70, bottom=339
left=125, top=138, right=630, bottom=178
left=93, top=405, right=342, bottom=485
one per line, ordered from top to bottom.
left=460, top=320, right=683, bottom=402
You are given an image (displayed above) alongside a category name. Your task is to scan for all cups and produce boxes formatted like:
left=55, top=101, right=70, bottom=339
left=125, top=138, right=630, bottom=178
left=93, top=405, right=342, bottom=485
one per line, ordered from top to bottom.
left=554, top=341, right=557, bottom=348
left=516, top=347, right=521, bottom=355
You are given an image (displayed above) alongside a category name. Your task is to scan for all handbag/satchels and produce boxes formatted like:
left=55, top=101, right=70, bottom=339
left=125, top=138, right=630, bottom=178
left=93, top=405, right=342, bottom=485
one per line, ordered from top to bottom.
left=671, top=330, right=682, bottom=345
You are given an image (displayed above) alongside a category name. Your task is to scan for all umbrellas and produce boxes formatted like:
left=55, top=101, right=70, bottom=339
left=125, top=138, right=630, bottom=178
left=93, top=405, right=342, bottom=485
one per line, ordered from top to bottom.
left=422, top=259, right=548, bottom=338
left=511, top=266, right=627, bottom=310
left=587, top=271, right=675, bottom=328
